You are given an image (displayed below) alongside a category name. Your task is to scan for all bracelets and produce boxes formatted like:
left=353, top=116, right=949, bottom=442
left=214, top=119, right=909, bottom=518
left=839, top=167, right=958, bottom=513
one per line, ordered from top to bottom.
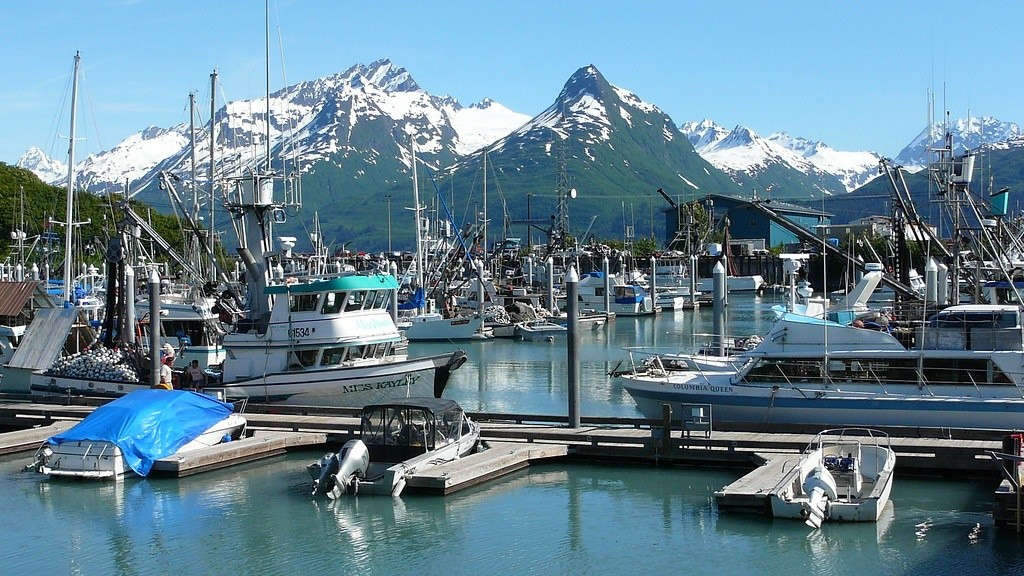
left=205, top=382, right=208, bottom=383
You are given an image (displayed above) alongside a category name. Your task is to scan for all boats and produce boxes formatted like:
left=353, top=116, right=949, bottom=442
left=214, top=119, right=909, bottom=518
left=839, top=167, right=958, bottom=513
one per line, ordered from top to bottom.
left=20, top=385, right=248, bottom=482
left=306, top=397, right=482, bottom=500
left=766, top=426, right=897, bottom=529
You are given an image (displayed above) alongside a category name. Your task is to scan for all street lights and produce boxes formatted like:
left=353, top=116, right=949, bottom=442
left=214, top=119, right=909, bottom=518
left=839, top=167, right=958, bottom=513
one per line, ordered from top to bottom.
left=646, top=192, right=655, bottom=239
left=384, top=194, right=394, bottom=256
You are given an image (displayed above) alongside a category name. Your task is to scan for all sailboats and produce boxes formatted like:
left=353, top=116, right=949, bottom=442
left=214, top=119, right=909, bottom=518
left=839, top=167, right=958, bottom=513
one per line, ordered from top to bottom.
left=1, top=0, right=1023, bottom=432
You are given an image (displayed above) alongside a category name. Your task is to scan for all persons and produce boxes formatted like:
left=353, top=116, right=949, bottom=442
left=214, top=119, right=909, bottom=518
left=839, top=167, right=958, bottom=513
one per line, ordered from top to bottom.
left=159, top=357, right=174, bottom=390
left=184, top=359, right=208, bottom=388
